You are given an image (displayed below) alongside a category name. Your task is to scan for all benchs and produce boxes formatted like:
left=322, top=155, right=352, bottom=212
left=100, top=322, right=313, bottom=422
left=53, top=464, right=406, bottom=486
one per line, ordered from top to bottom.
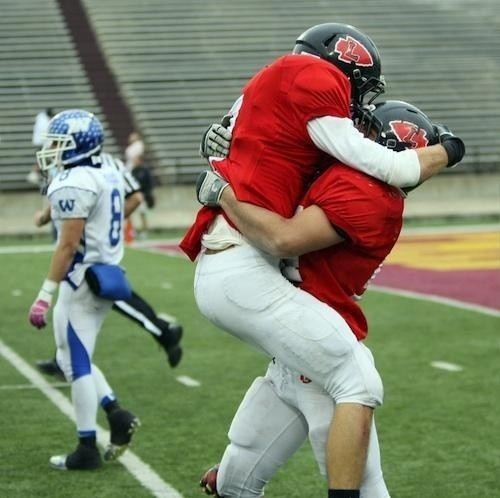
left=0, top=0, right=500, bottom=195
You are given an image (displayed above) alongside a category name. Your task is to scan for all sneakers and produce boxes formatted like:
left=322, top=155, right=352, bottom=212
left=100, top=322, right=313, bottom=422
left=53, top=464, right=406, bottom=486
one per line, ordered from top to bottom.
left=166, top=325, right=182, bottom=367
left=36, top=360, right=58, bottom=373
left=105, top=409, right=139, bottom=462
left=49, top=450, right=102, bottom=470
left=200, top=464, right=225, bottom=498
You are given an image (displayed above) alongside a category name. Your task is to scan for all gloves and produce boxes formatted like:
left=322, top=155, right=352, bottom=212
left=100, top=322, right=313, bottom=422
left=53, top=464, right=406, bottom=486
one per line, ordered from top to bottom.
left=200, top=114, right=232, bottom=158
left=432, top=120, right=465, bottom=169
left=196, top=170, right=230, bottom=207
left=28, top=289, right=53, bottom=329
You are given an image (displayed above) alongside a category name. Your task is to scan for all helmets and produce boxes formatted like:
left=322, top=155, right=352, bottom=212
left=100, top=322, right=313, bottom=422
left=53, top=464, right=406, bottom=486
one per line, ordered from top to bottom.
left=292, top=22, right=386, bottom=105
left=352, top=100, right=438, bottom=198
left=36, top=109, right=105, bottom=171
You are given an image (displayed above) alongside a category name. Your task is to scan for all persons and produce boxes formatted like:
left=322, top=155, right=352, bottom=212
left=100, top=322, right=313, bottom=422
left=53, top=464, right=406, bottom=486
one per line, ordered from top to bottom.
left=180, top=21, right=466, bottom=498
left=195, top=97, right=437, bottom=498
left=22, top=99, right=157, bottom=249
left=28, top=107, right=140, bottom=472
left=31, top=149, right=183, bottom=376
